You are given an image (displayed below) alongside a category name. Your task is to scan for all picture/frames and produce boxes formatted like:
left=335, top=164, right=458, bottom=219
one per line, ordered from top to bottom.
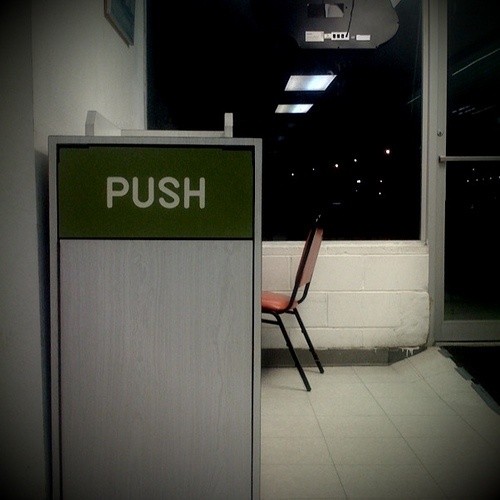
left=104, top=0, right=137, bottom=47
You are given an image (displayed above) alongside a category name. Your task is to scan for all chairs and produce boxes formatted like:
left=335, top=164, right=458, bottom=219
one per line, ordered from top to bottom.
left=263, top=213, right=327, bottom=394
